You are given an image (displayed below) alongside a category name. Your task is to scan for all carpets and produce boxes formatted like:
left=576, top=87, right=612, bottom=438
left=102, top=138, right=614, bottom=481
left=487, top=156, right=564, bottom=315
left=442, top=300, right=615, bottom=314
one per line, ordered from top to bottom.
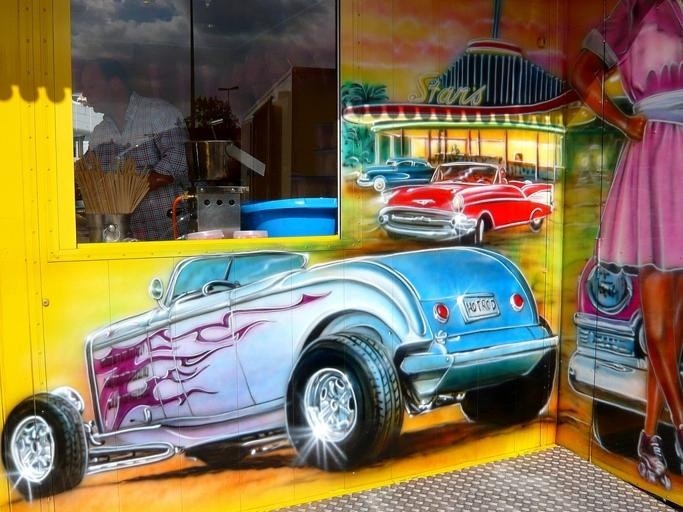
left=272, top=444, right=682, bottom=511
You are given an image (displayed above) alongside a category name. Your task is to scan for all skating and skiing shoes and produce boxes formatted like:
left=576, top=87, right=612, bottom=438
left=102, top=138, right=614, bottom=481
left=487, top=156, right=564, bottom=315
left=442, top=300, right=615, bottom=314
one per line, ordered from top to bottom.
left=635, top=430, right=671, bottom=490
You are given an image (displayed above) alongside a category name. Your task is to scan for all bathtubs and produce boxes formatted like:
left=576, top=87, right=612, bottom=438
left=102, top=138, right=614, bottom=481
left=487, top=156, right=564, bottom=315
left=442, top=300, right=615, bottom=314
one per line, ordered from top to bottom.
left=238, top=195, right=338, bottom=238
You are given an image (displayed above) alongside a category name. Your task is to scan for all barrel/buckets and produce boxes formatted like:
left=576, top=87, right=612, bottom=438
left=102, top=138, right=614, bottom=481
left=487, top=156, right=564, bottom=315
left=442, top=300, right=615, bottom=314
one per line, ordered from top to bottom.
left=184, top=126, right=235, bottom=183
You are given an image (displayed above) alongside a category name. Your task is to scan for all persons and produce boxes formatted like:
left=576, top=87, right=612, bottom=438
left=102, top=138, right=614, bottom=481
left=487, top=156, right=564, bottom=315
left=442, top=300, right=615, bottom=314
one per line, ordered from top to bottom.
left=568, top=0, right=683, bottom=477
left=79, top=57, right=194, bottom=241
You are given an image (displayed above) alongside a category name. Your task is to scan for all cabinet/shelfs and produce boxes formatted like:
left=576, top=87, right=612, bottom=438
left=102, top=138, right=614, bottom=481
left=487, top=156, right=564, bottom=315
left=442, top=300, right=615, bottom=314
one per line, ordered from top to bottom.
left=239, top=65, right=336, bottom=199
